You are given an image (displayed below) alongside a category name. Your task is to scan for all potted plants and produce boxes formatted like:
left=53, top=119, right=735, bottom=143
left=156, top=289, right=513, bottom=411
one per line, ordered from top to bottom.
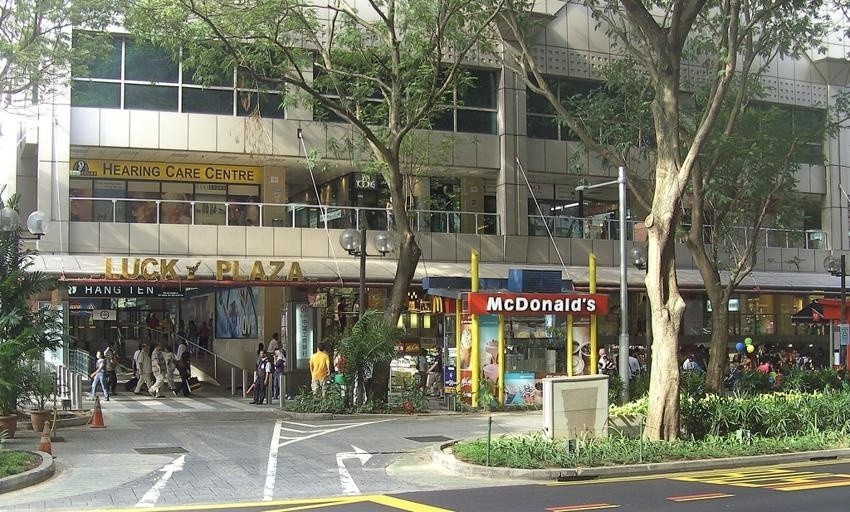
left=0, top=191, right=80, bottom=441
left=14, top=366, right=61, bottom=433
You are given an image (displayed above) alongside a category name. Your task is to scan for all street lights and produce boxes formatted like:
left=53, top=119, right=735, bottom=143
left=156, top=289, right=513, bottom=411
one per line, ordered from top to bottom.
left=0, top=206, right=50, bottom=417
left=338, top=224, right=397, bottom=412
left=631, top=240, right=651, bottom=380
left=823, top=251, right=850, bottom=370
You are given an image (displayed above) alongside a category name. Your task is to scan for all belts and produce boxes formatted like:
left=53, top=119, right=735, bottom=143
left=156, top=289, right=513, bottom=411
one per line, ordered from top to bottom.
left=106, top=371, right=115, bottom=373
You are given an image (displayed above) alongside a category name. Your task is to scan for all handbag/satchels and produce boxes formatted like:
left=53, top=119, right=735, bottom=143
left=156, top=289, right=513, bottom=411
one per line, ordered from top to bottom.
left=631, top=370, right=638, bottom=377
left=188, top=376, right=201, bottom=391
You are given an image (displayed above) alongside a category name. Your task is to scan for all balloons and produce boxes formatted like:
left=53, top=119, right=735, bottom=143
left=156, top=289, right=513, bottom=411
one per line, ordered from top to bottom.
left=737, top=338, right=755, bottom=353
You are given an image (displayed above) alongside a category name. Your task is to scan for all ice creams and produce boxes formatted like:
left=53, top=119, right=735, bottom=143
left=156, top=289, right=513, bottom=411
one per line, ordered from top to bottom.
left=460, top=327, right=472, bottom=369
left=485, top=339, right=499, bottom=363
left=483, top=363, right=499, bottom=397
left=503, top=383, right=518, bottom=403
left=518, top=383, right=535, bottom=405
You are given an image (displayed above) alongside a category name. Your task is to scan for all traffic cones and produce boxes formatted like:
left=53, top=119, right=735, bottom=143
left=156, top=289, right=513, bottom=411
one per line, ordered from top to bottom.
left=88, top=396, right=107, bottom=428
left=37, top=421, right=57, bottom=460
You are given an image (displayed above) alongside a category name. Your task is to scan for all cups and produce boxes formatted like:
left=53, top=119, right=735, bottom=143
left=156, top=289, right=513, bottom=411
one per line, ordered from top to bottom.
left=459, top=331, right=471, bottom=368
left=480, top=340, right=499, bottom=396
left=572, top=340, right=592, bottom=376
left=505, top=382, right=543, bottom=404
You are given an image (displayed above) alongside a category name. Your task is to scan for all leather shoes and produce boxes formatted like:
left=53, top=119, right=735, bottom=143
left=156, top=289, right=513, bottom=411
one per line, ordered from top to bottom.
left=249, top=400, right=257, bottom=404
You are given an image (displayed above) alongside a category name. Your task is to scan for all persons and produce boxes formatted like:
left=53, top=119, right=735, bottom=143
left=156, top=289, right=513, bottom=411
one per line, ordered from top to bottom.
left=309, top=298, right=360, bottom=397
left=86, top=312, right=214, bottom=398
left=250, top=333, right=288, bottom=405
left=597, top=344, right=823, bottom=383
left=412, top=345, right=444, bottom=399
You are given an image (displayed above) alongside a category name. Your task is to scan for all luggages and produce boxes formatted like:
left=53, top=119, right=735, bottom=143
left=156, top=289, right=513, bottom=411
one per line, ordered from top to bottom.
left=125, top=377, right=139, bottom=392
